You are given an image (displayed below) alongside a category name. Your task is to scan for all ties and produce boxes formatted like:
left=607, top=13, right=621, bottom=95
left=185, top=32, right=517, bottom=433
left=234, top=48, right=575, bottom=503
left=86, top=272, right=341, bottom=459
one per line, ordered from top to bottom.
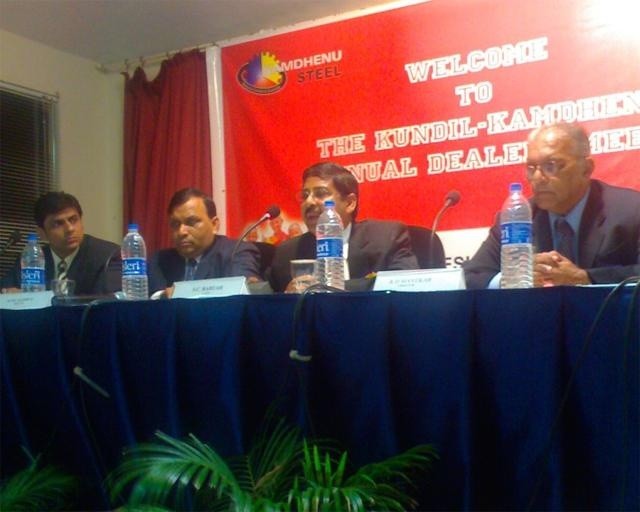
left=554, top=216, right=575, bottom=265
left=186, top=258, right=198, bottom=280
left=55, top=260, right=68, bottom=297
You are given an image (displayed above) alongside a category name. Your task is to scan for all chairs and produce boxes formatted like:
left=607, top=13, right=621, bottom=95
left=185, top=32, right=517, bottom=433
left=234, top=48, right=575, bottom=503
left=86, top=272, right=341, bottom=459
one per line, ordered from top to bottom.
left=103, top=224, right=446, bottom=294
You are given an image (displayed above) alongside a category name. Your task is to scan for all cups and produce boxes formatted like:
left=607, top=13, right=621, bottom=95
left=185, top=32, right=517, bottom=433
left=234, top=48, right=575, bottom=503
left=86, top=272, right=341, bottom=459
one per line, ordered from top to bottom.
left=289, top=257, right=317, bottom=295
left=50, top=279, right=77, bottom=306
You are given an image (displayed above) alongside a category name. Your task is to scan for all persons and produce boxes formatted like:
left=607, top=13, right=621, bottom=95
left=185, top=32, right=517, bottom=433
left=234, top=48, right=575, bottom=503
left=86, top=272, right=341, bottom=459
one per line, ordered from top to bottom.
left=460, top=121, right=640, bottom=291
left=269, top=162, right=424, bottom=296
left=144, top=188, right=263, bottom=299
left=0, top=189, right=123, bottom=296
left=264, top=214, right=289, bottom=244
left=245, top=223, right=259, bottom=241
left=287, top=225, right=303, bottom=239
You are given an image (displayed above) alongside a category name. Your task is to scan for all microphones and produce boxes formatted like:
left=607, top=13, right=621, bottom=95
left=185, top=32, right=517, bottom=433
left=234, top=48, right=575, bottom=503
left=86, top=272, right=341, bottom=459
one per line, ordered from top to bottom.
left=428, top=190, right=461, bottom=269
left=228, top=206, right=281, bottom=278
left=0, top=230, right=21, bottom=256
left=51, top=291, right=125, bottom=308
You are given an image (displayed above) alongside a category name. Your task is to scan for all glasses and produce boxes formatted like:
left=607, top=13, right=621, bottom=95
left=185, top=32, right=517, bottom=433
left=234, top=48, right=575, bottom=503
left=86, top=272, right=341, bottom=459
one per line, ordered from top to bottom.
left=526, top=159, right=577, bottom=181
left=296, top=188, right=343, bottom=202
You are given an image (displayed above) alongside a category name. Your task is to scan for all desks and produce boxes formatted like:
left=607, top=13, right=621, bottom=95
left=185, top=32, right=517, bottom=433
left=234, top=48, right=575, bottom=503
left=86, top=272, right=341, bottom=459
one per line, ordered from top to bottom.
left=0, top=275, right=638, bottom=511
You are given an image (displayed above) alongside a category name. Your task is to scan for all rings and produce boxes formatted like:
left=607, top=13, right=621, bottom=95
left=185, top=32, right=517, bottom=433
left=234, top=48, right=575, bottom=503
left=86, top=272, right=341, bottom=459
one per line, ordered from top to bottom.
left=546, top=266, right=553, bottom=273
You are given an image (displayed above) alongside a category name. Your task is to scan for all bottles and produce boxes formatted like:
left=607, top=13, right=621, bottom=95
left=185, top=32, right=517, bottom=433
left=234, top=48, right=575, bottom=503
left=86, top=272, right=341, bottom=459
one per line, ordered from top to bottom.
left=500, top=184, right=534, bottom=289
left=314, top=200, right=346, bottom=295
left=120, top=224, right=149, bottom=301
left=20, top=234, right=46, bottom=294
